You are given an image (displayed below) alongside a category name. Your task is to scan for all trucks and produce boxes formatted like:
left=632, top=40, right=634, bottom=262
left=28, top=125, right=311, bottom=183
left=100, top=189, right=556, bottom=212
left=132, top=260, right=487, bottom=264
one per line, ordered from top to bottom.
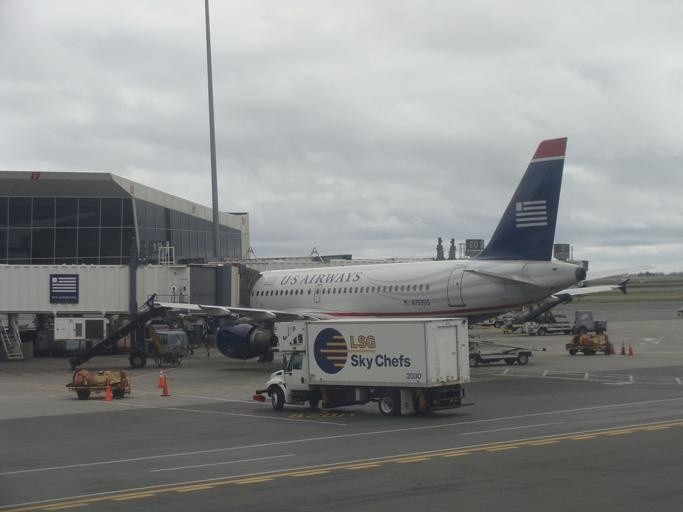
left=524, top=309, right=598, bottom=336
left=254, top=316, right=473, bottom=420
left=469, top=338, right=547, bottom=368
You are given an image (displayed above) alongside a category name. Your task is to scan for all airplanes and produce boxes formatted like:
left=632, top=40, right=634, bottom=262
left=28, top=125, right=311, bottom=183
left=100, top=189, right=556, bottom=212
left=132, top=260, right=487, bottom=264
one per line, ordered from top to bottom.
left=151, top=132, right=660, bottom=369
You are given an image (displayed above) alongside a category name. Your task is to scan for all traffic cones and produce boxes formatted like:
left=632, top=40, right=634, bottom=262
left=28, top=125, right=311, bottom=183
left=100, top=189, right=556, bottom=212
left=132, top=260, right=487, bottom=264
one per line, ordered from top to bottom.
left=619, top=342, right=628, bottom=355
left=627, top=343, right=636, bottom=356
left=158, top=370, right=168, bottom=388
left=159, top=378, right=171, bottom=397
left=105, top=382, right=114, bottom=402
